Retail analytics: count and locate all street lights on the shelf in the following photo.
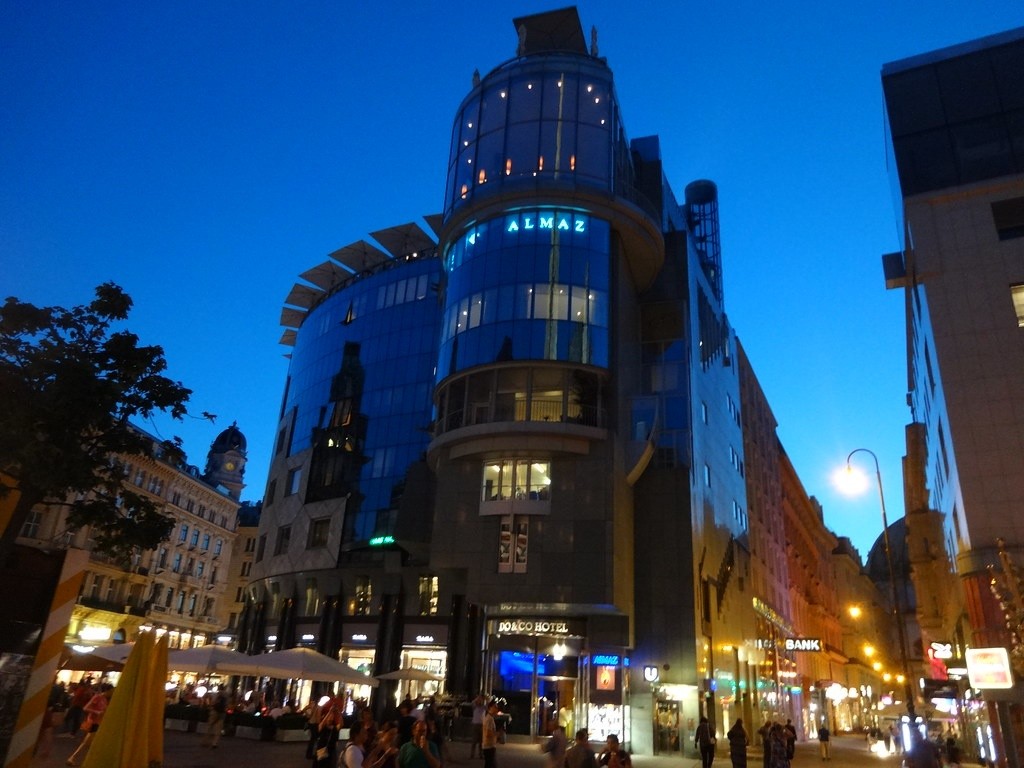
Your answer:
[838,448,922,751]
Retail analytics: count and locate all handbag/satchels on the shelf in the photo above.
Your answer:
[707,723,717,744]
[787,750,793,759]
[317,747,329,761]
[88,723,99,732]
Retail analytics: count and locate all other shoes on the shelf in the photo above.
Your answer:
[827,758,830,761]
[65,761,81,766]
[212,746,217,749]
[822,758,825,761]
[200,743,209,748]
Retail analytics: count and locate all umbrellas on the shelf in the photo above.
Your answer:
[59,642,443,687]
[81,628,165,768]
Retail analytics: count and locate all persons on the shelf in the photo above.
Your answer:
[863,723,944,754]
[202,684,499,768]
[51,679,115,768]
[694,716,797,768]
[540,704,632,768]
[818,724,832,761]
